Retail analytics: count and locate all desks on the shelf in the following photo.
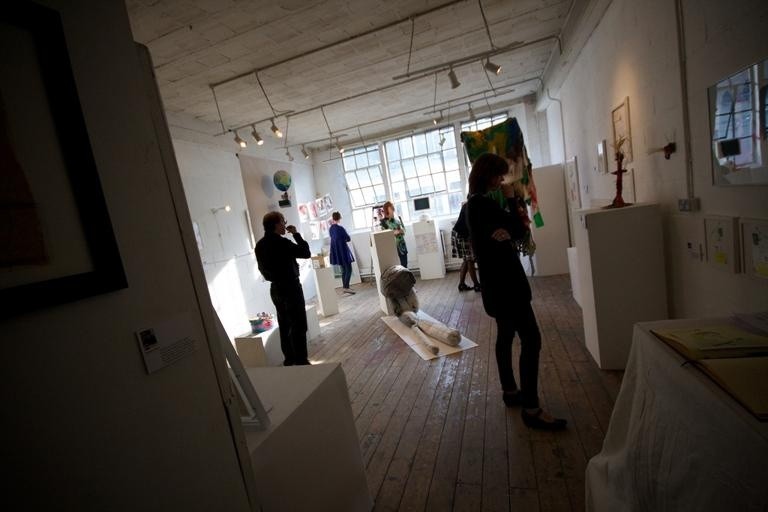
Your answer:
[627,315,766,510]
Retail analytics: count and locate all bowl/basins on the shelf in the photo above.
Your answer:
[249,316,273,335]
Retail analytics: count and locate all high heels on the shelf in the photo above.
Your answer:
[521,410,567,433]
[503,390,525,408]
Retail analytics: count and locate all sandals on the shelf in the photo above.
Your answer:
[474,284,481,292]
[458,283,474,292]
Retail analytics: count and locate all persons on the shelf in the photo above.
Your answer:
[450,203,481,292]
[254,211,311,366]
[329,212,356,294]
[380,202,408,268]
[464,154,567,431]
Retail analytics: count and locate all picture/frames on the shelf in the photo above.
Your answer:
[0,0,130,329]
[610,95,633,164]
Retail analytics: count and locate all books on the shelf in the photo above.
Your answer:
[649,326,768,423]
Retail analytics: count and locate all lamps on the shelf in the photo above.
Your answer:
[431,100,482,129]
[206,68,294,148]
[278,104,350,162]
[436,121,450,147]
[442,1,503,89]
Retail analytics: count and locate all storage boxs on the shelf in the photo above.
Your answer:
[312,255,328,268]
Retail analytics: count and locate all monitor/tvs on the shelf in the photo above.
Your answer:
[412,196,431,212]
